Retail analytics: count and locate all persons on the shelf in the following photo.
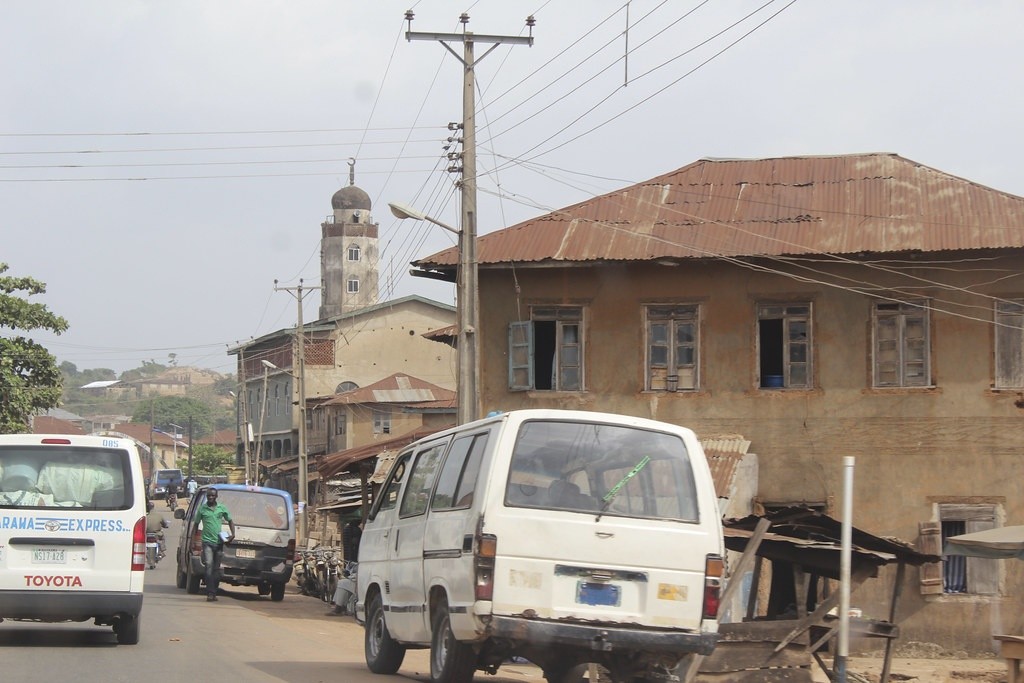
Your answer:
[189,487,235,602]
[164,477,179,507]
[145,502,169,558]
[325,530,362,617]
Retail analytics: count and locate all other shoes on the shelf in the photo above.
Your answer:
[324,605,342,616]
[213,593,219,601]
[207,593,214,602]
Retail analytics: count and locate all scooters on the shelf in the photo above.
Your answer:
[293,542,358,615]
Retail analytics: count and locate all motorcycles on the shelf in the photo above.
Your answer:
[165,488,178,511]
[145,520,172,570]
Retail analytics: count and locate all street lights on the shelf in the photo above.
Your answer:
[262,357,309,540]
[390,198,474,428]
[228,391,250,484]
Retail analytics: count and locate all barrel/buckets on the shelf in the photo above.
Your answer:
[764,375,784,387]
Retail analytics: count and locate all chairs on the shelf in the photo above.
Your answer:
[0,476,34,492]
[91,489,124,508]
[545,480,580,507]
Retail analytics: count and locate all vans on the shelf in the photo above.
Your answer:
[174,483,297,602]
[0,432,154,645]
[146,469,183,499]
[339,407,729,683]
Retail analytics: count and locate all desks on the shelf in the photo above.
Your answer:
[994,634,1024,683]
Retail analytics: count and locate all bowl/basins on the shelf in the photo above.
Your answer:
[850,608,862,617]
